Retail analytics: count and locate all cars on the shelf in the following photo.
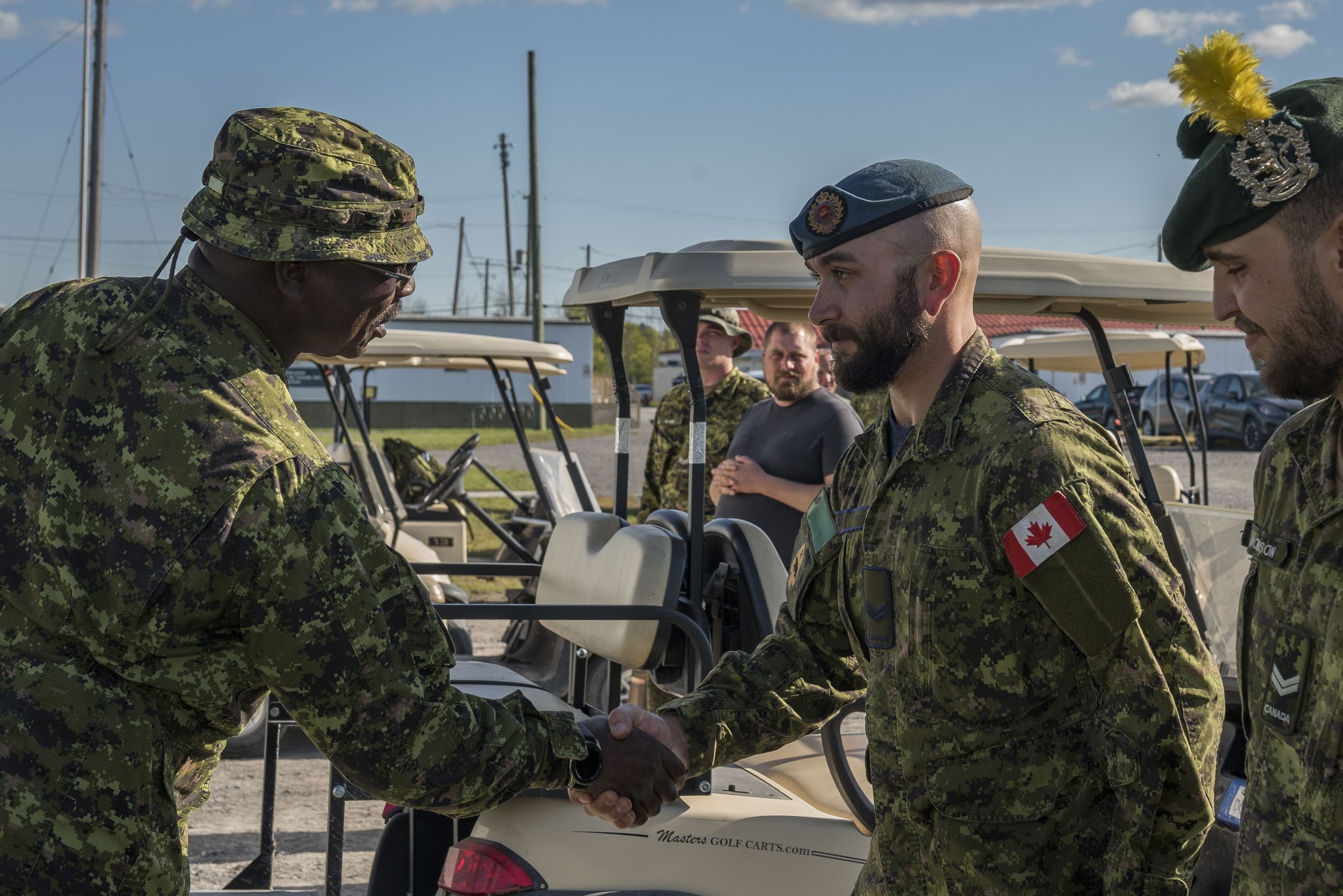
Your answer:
[1071,384,1148,430]
[1138,372,1217,436]
[1185,370,1305,451]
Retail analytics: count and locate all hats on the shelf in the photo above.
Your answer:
[181,107,434,265]
[697,305,754,359]
[1159,31,1343,273]
[790,159,975,259]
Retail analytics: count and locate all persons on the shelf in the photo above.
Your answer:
[1163,30,1343,896]
[635,291,889,570]
[568,160,1226,895]
[0,108,685,896]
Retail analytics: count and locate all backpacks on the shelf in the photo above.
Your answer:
[383,437,447,505]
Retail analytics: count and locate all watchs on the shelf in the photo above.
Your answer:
[570,721,605,793]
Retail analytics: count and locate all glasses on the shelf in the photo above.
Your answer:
[343,261,418,290]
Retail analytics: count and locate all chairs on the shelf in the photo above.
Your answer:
[445,505,790,724]
[734,699,882,840]
[330,439,469,565]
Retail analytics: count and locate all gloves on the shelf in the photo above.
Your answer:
[571,714,688,828]
